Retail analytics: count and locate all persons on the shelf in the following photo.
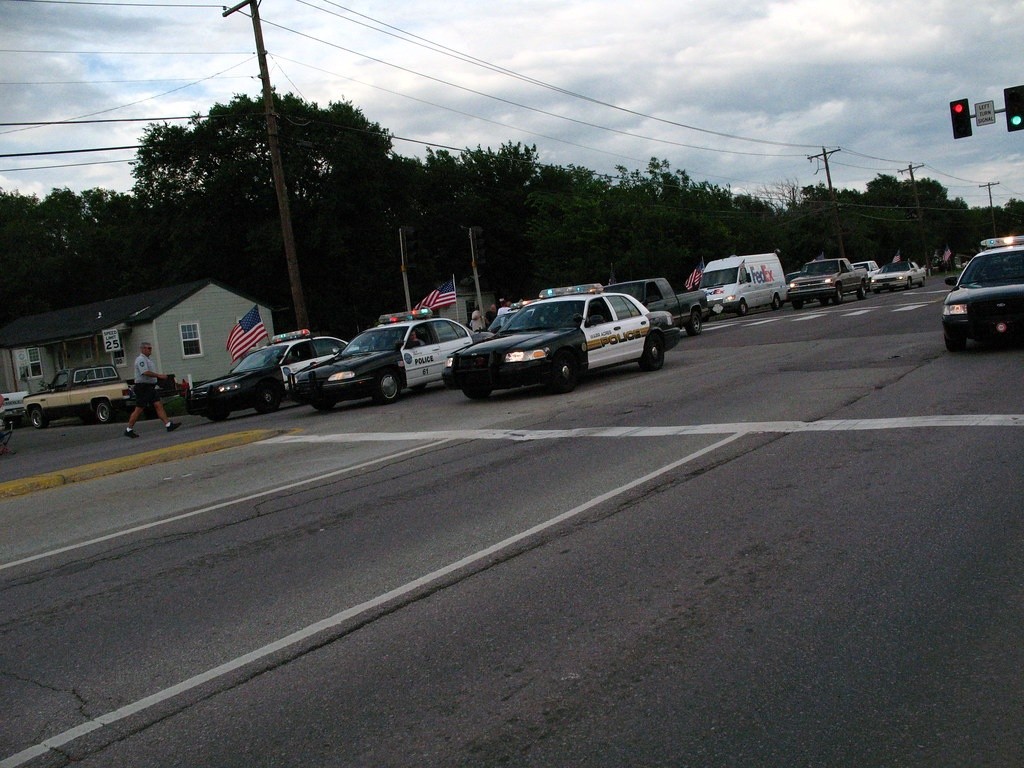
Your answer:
[123,339,182,438]
[469,300,512,331]
[408,329,425,346]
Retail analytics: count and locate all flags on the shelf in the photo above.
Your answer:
[684,260,705,291]
[413,279,456,311]
[942,244,951,263]
[891,250,900,263]
[226,304,268,365]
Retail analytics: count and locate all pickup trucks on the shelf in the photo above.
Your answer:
[0,390,29,427]
[23,364,178,429]
[603,277,708,336]
[787,258,868,309]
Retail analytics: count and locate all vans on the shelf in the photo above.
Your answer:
[700,252,788,318]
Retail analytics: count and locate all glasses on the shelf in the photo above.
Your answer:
[144,347,151,349]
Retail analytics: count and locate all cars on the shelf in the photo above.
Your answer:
[943,245,1024,351]
[851,261,881,291]
[188,336,371,422]
[296,318,495,410]
[442,293,681,399]
[784,271,801,302]
[869,261,926,293]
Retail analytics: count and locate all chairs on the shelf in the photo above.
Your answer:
[384,336,399,347]
[418,333,431,345]
[982,266,1004,282]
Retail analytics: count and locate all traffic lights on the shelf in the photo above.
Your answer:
[1004,85,1024,132]
[950,98,973,139]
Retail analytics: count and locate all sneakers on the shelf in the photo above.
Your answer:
[167,422,182,432]
[124,430,139,438]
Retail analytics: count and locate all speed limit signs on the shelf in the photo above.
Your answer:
[102,330,121,352]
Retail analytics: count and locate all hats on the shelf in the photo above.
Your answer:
[499,298,507,302]
